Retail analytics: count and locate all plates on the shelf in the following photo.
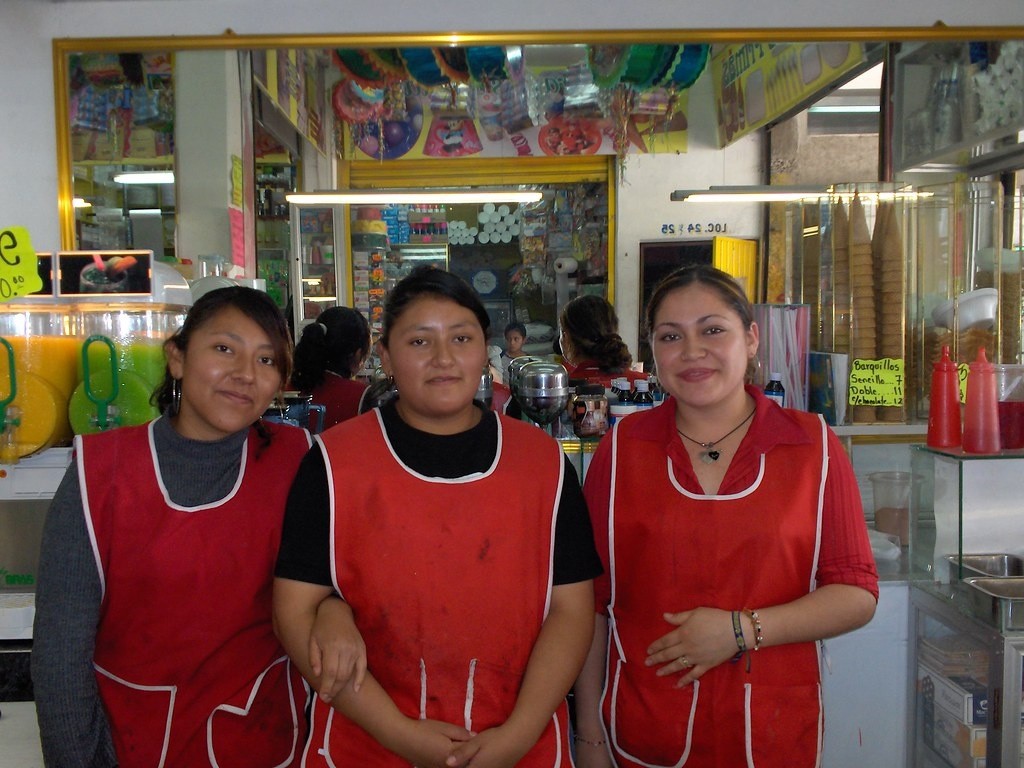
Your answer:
[973,247,1020,273]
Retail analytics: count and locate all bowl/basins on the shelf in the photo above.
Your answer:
[931,288,999,335]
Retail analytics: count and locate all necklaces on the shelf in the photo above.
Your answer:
[676,408,756,465]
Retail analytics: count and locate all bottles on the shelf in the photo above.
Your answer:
[962,346,1000,454]
[615,380,668,407]
[927,345,962,448]
[611,377,627,393]
[764,372,785,408]
[255,173,290,216]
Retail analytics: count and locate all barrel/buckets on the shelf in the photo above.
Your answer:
[320,245,333,264]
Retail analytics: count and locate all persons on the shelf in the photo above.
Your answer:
[580,264,879,768]
[269,263,605,768]
[30,286,368,768]
[280,294,653,442]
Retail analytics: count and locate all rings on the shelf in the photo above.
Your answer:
[682,655,691,669]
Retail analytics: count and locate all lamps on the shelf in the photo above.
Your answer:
[285,190,543,205]
[669,184,920,204]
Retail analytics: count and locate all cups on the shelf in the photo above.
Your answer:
[198,255,222,277]
[79,261,128,293]
[868,471,924,546]
[993,365,1024,449]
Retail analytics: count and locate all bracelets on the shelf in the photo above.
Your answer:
[732,609,762,672]
